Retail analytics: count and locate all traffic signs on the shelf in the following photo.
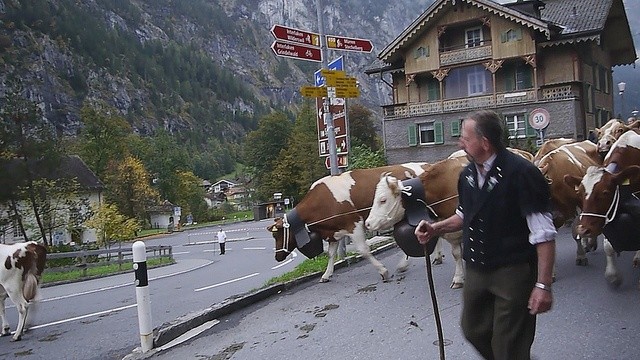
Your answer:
[529,108,551,130]
[313,55,350,169]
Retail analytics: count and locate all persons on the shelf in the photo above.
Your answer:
[619,119,625,124]
[415,109,559,360]
[214,228,227,255]
[628,117,635,125]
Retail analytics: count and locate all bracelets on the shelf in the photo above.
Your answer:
[535,282,552,291]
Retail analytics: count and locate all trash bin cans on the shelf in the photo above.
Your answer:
[254,204,267,221]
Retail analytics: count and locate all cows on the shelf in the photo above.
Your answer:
[533,118,640,290]
[364,144,536,290]
[0,239,46,340]
[266,160,445,284]
[446,148,534,162]
